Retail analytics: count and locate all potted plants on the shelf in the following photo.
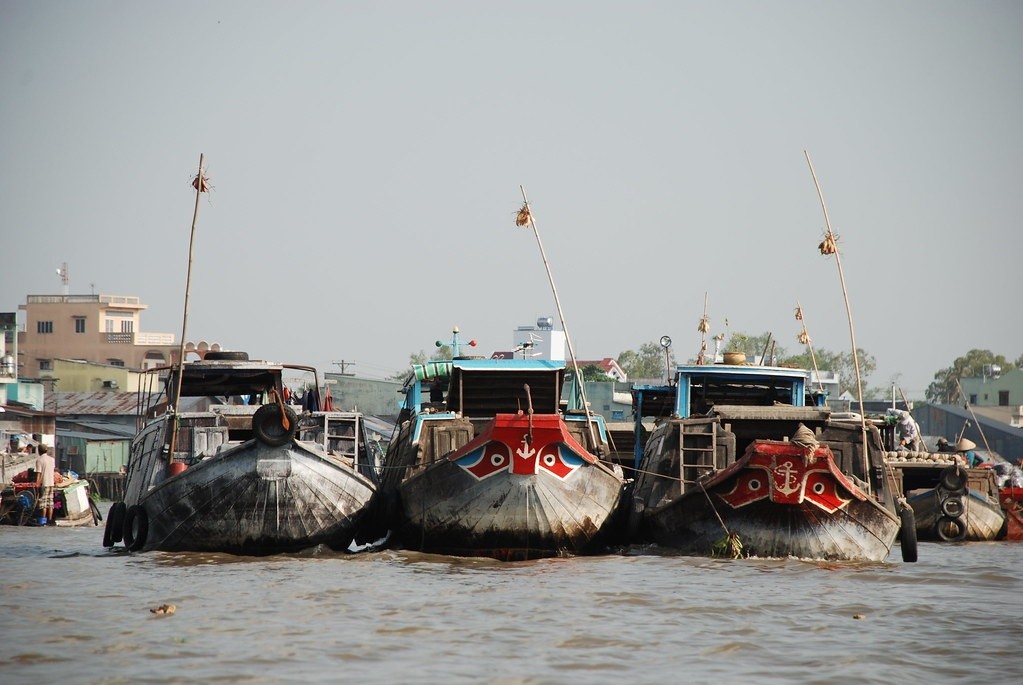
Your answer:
[723,318,748,365]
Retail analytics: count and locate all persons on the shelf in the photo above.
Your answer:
[36,444,55,525]
[935,438,984,470]
[886,408,920,451]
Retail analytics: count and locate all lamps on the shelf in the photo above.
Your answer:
[660,336,674,413]
[103,380,121,390]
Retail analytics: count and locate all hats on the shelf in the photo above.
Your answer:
[38,444,48,451]
[935,438,948,446]
[956,438,977,451]
[899,411,910,425]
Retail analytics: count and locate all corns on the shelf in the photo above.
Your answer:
[796,311,808,344]
[697,322,708,332]
[516,214,530,227]
[818,238,835,254]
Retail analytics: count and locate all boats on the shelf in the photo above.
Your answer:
[0,437,101,527]
[100,142,382,551]
[360,179,638,560]
[628,150,902,561]
[885,458,1005,544]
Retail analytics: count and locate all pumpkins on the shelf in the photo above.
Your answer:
[883,450,961,465]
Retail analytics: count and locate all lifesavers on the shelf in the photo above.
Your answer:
[898,509,918,563]
[934,465,970,541]
[250,402,300,447]
[102,501,150,550]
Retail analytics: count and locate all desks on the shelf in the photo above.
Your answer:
[874,420,896,452]
